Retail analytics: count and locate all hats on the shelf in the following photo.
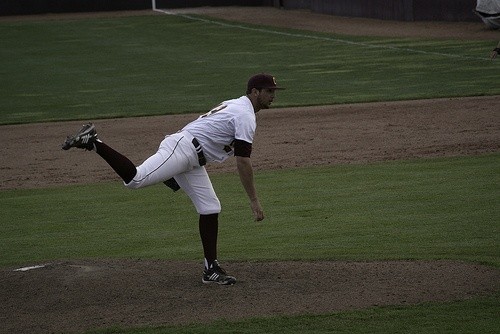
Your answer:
[248,72,286,89]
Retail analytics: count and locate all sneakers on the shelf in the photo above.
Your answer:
[201,257,237,286]
[62,121,98,151]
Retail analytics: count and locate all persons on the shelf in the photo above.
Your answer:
[62,73,286,285]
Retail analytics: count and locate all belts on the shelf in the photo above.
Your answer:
[192,138,206,166]
